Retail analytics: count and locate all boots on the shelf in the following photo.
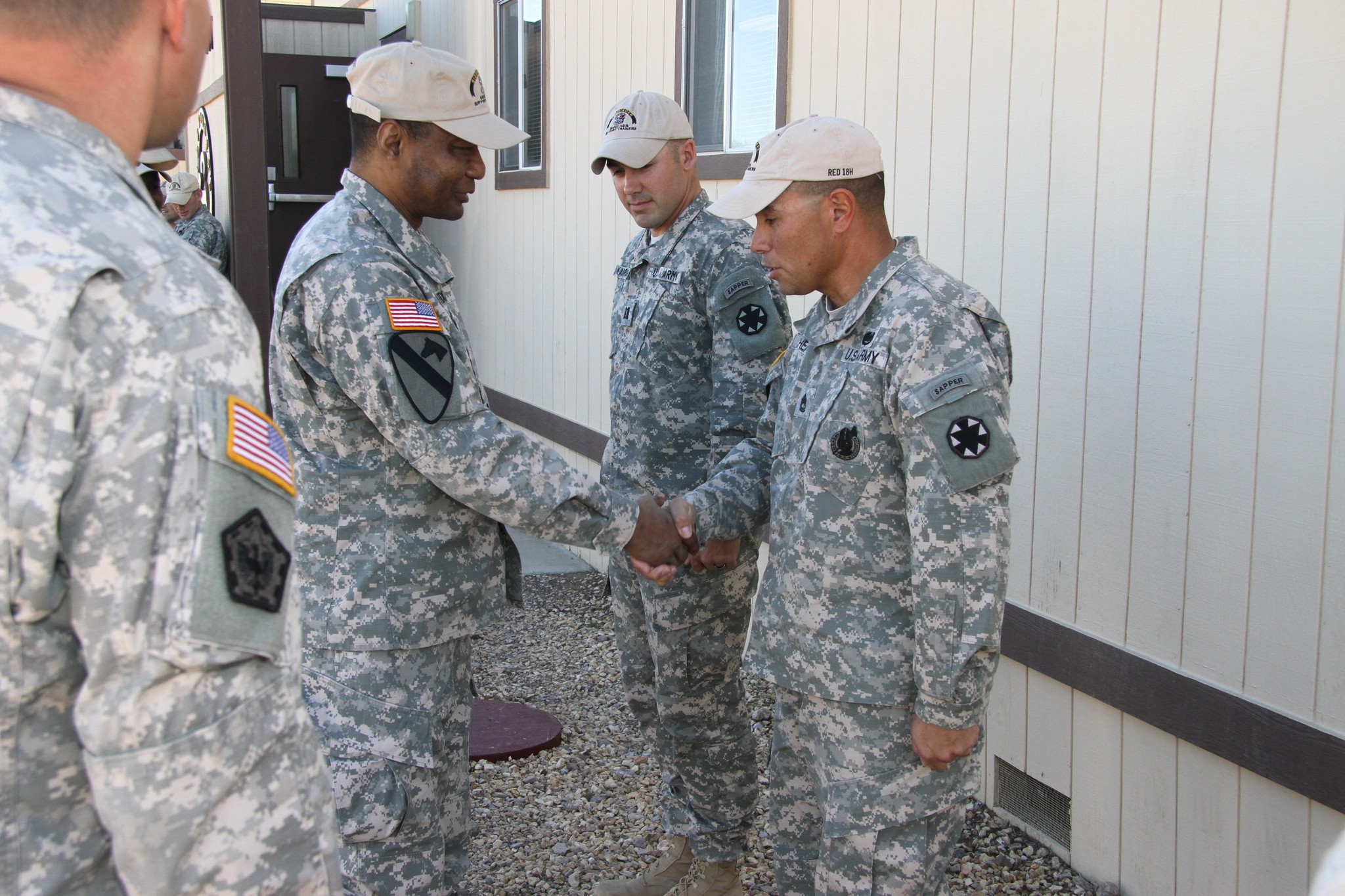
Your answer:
[593,828,693,896]
[661,859,743,896]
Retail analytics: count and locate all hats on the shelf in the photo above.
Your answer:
[344,40,536,148]
[708,116,884,220]
[586,89,693,175]
[164,173,198,206]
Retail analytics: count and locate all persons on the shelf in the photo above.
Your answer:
[627,113,1024,896]
[267,40,702,896]
[164,170,227,278]
[0,0,349,896]
[134,160,173,213]
[157,180,183,234]
[585,84,801,893]
